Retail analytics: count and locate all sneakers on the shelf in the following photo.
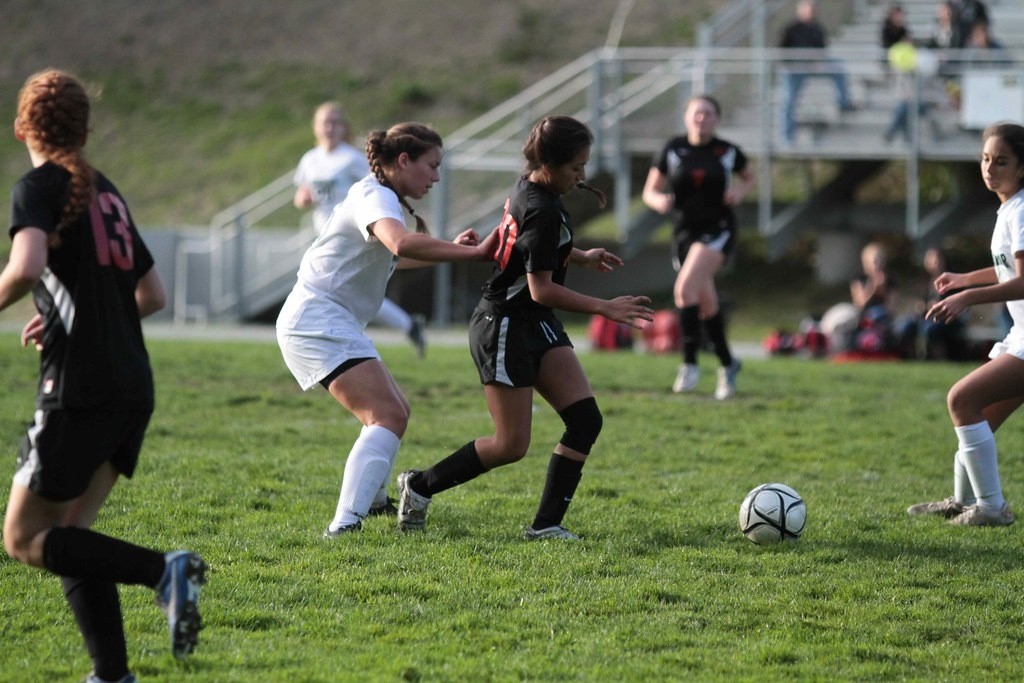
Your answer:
[396,468,433,532]
[714,358,740,400]
[673,362,700,392]
[949,504,1014,527]
[156,548,209,658]
[906,497,965,519]
[82,670,135,683]
[523,523,579,540]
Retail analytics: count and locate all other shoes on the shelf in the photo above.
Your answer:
[367,495,398,516]
[323,521,362,539]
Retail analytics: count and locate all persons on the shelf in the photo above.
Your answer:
[910,120,1022,527]
[404,115,656,546]
[0,67,211,683]
[276,122,499,542]
[297,0,1022,404]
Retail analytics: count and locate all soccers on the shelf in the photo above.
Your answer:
[738,482,807,547]
